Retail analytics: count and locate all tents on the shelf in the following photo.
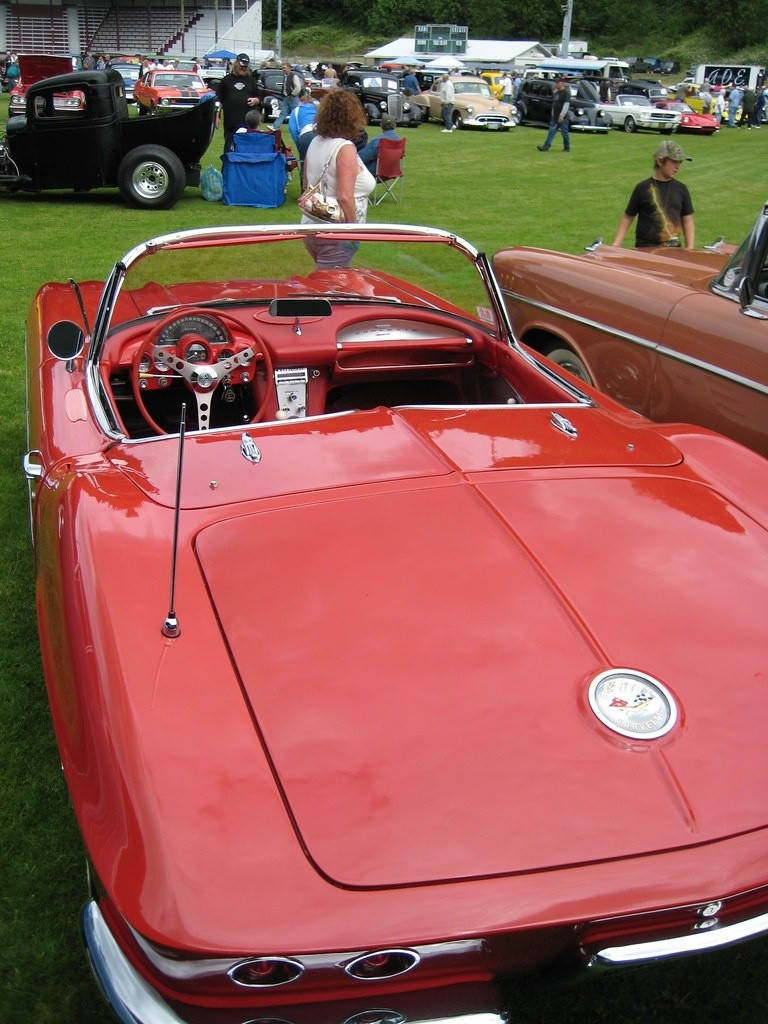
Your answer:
[203,50,239,70]
[383,54,426,66]
[425,56,464,68]
[229,49,282,72]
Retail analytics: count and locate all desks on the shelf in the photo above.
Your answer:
[293,160,304,178]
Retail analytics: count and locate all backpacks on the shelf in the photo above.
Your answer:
[290,72,306,94]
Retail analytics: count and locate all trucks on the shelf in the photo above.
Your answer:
[682,64,766,94]
[537,58,631,82]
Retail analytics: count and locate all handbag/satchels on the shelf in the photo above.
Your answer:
[281,140,298,171]
[200,165,223,202]
[297,184,350,225]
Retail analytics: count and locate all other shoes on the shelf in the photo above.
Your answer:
[537,146,549,152]
[451,125,457,129]
[722,123,763,131]
[560,149,570,153]
[441,129,452,133]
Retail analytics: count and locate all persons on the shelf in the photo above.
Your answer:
[300,92,376,269]
[4,51,19,93]
[82,51,112,71]
[441,74,457,133]
[214,53,264,173]
[447,67,482,77]
[387,65,421,96]
[305,64,311,72]
[597,79,611,104]
[142,57,233,76]
[324,63,349,78]
[496,71,568,105]
[537,78,570,152]
[288,95,402,194]
[231,110,293,194]
[266,63,301,131]
[612,140,695,249]
[675,78,768,130]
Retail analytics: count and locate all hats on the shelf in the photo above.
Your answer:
[410,69,415,74]
[653,141,695,162]
[175,58,181,62]
[553,76,562,80]
[236,53,250,67]
[381,115,396,129]
[694,78,767,93]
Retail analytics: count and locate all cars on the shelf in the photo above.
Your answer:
[57,53,227,91]
[253,69,321,123]
[338,70,423,128]
[133,70,221,117]
[411,76,520,132]
[604,56,681,75]
[9,53,86,118]
[595,94,682,135]
[109,64,142,100]
[523,69,624,100]
[291,61,514,100]
[618,79,768,124]
[491,201,767,461]
[1,69,218,210]
[29,221,767,1023]
[514,78,613,134]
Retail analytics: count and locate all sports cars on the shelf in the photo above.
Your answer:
[651,101,722,135]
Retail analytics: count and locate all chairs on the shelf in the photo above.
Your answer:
[220,132,295,208]
[624,101,633,106]
[368,137,407,207]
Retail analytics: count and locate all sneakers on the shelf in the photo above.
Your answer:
[267,125,276,132]
